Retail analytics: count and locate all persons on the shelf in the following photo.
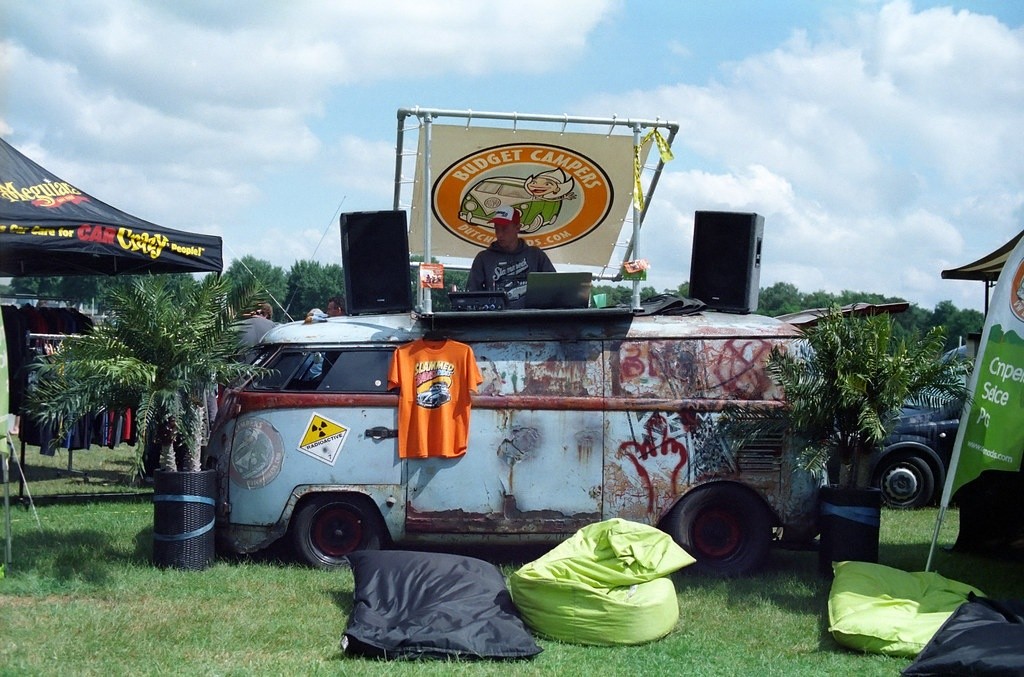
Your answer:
[465,206,557,309]
[326,297,345,317]
[237,302,277,348]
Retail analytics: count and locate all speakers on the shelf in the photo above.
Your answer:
[689,211,765,313]
[340,210,412,316]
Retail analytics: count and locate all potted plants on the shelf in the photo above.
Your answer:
[762,297,975,579]
[28,263,280,571]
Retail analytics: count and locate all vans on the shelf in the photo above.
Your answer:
[199,302,910,572]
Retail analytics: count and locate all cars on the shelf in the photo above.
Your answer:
[827,340,967,512]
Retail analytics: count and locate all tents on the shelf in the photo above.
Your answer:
[0,137,223,331]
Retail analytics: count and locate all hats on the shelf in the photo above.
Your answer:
[487,205,522,227]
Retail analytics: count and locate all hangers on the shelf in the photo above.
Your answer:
[422,314,447,342]
[34,333,66,353]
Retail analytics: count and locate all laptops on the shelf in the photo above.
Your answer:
[524,272,592,311]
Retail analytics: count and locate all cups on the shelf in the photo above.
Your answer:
[593,294,606,308]
